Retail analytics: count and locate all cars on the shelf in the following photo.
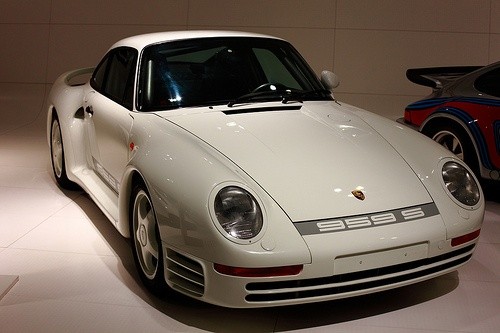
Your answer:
[397,59,499,182]
[45,29,486,309]
